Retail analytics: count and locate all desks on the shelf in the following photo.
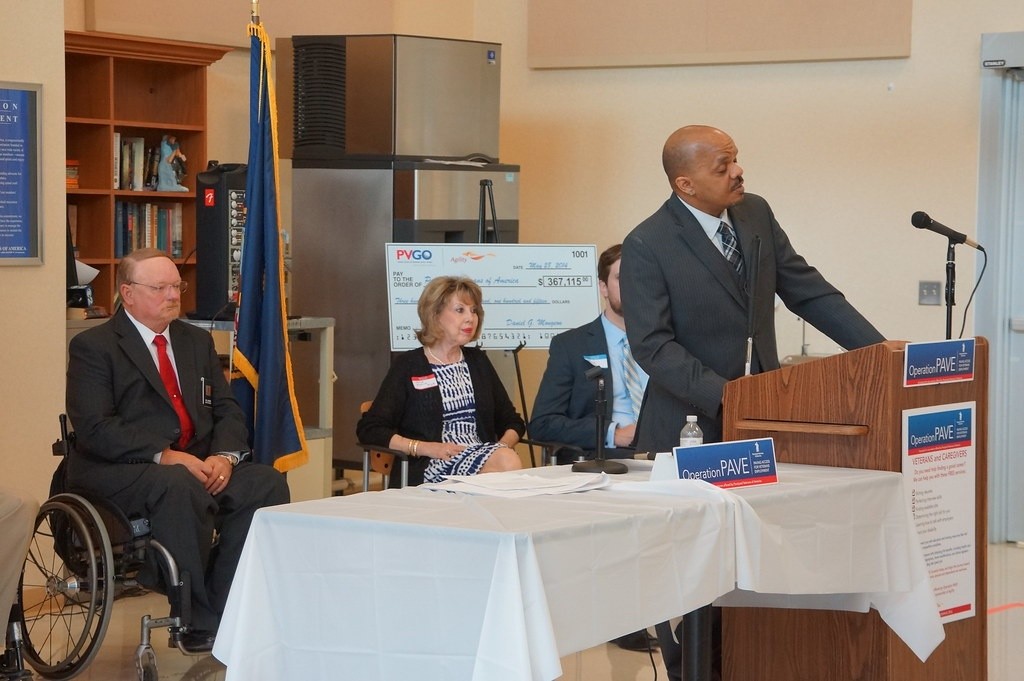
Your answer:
[257,458,903,681]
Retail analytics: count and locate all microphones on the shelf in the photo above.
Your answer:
[911,211,985,252]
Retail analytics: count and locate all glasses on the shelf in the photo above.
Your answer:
[128,281,188,294]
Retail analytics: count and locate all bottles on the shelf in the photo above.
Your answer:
[680,415,704,449]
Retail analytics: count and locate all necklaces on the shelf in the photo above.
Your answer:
[428,346,462,364]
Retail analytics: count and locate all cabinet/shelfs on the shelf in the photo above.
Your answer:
[66,317,336,582]
[64,30,234,319]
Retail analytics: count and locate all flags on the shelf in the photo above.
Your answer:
[233,21,309,474]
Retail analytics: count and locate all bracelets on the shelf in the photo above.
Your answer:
[408,440,420,456]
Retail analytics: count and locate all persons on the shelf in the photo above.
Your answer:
[620,125,912,681]
[527,244,659,653]
[65,247,287,655]
[158,135,186,188]
[356,276,524,490]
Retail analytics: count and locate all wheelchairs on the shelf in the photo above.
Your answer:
[14,412,222,681]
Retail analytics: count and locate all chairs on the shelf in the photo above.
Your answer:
[361,400,408,492]
[542,444,552,466]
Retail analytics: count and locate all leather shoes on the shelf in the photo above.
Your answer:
[167,629,217,651]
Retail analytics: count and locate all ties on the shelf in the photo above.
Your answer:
[152,335,196,453]
[622,336,645,424]
[717,220,744,275]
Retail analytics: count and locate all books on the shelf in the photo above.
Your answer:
[68,205,77,248]
[115,132,159,191]
[66,160,80,188]
[115,201,182,258]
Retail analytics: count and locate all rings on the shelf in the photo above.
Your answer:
[219,475,224,480]
[446,449,449,454]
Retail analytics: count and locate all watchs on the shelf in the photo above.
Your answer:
[221,454,237,466]
